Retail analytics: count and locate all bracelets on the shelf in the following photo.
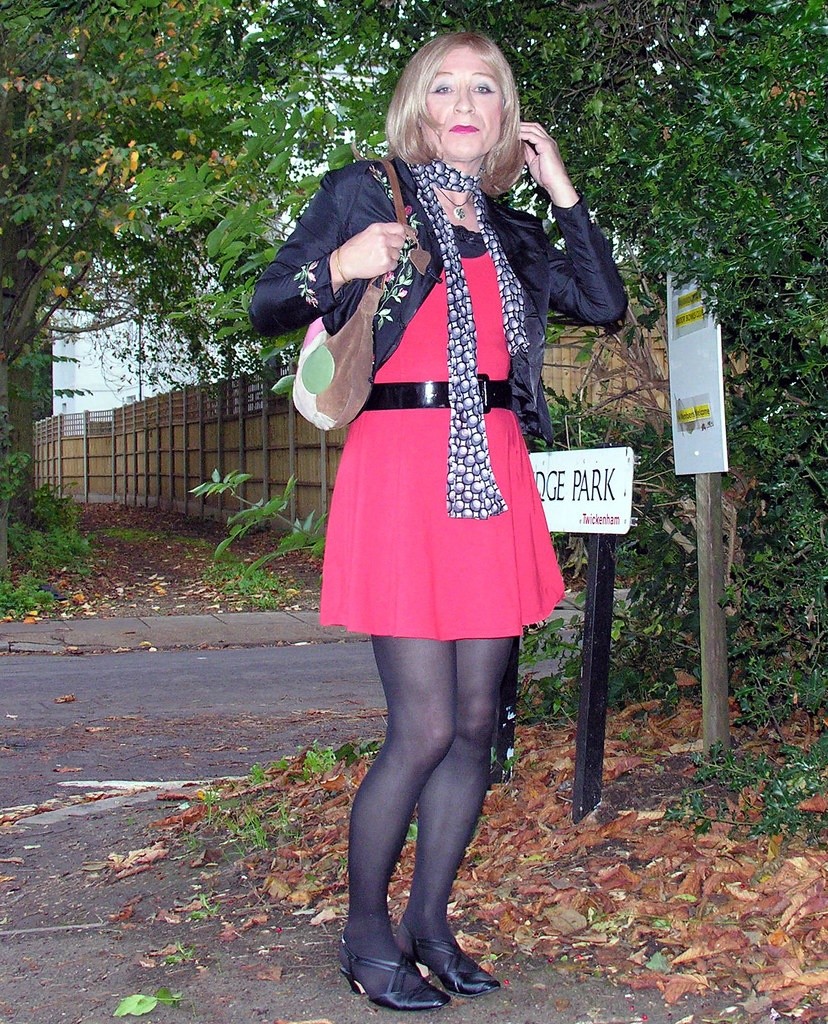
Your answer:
[335,246,354,285]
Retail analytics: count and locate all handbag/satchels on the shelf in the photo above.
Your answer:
[293,282,386,430]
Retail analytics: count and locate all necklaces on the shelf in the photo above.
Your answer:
[432,184,474,221]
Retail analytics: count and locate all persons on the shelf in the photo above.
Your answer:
[245,30,631,1013]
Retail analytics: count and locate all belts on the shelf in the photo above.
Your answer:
[364,374,513,413]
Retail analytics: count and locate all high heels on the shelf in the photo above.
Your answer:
[401,916,500,996]
[340,936,452,1010]
